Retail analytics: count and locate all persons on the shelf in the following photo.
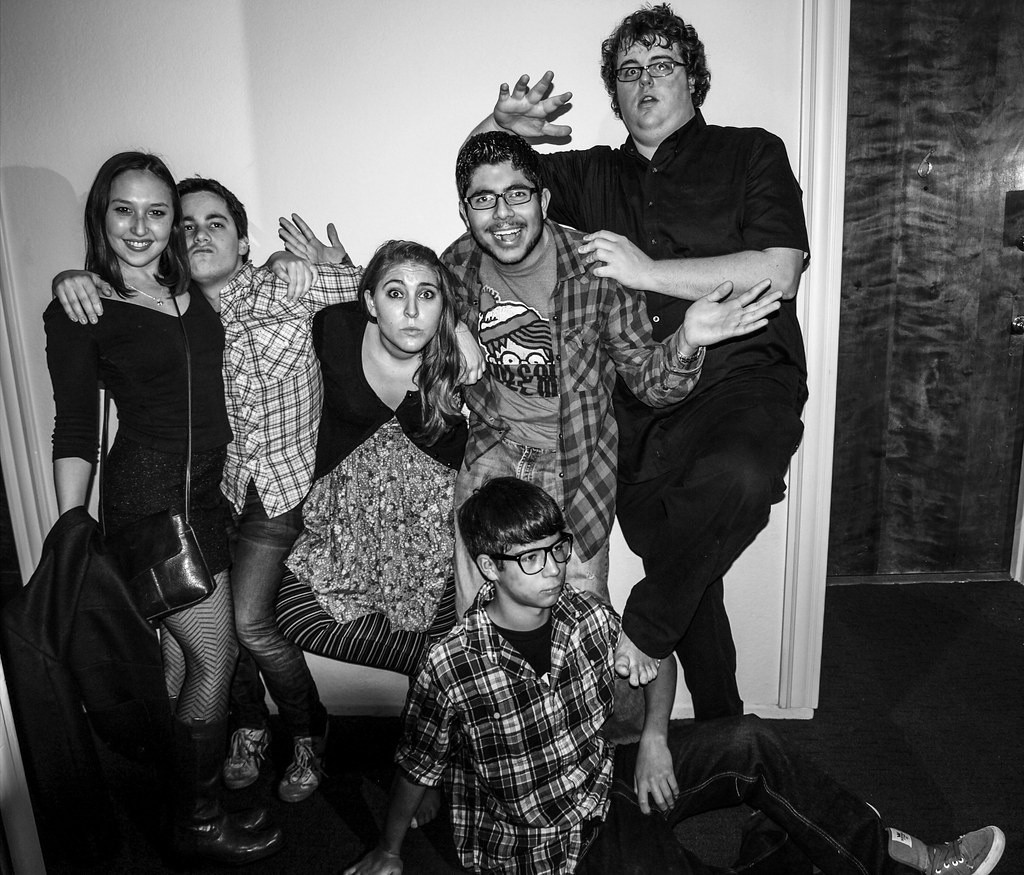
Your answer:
[43,4,1007,875]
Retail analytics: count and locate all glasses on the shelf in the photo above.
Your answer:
[490,532,574,576]
[615,61,687,82]
[463,188,538,210]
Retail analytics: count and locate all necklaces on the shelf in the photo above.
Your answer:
[120,278,165,307]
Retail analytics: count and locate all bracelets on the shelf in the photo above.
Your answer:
[676,345,704,365]
[376,844,400,859]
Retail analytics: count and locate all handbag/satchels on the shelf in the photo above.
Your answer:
[104,508,217,621]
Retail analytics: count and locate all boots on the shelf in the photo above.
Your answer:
[160,712,285,867]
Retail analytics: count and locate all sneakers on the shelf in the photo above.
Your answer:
[886,825,1006,875]
[278,717,330,803]
[750,798,882,821]
[222,727,271,790]
